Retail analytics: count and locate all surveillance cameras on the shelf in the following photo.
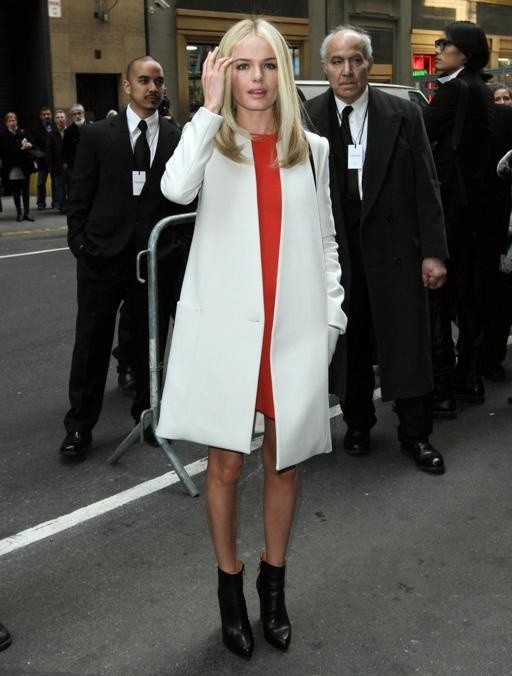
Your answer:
[154,0,170,9]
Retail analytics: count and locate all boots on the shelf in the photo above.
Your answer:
[217,562,254,660]
[257,552,292,653]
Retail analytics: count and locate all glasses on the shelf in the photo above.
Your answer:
[434,38,454,51]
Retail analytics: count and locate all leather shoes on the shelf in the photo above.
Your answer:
[59,429,94,457]
[117,364,141,391]
[399,439,445,474]
[343,425,373,455]
[434,390,457,420]
[460,370,486,403]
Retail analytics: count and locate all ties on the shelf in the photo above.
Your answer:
[133,123,151,170]
[339,107,362,230]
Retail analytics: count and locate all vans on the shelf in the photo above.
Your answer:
[293,77,433,112]
